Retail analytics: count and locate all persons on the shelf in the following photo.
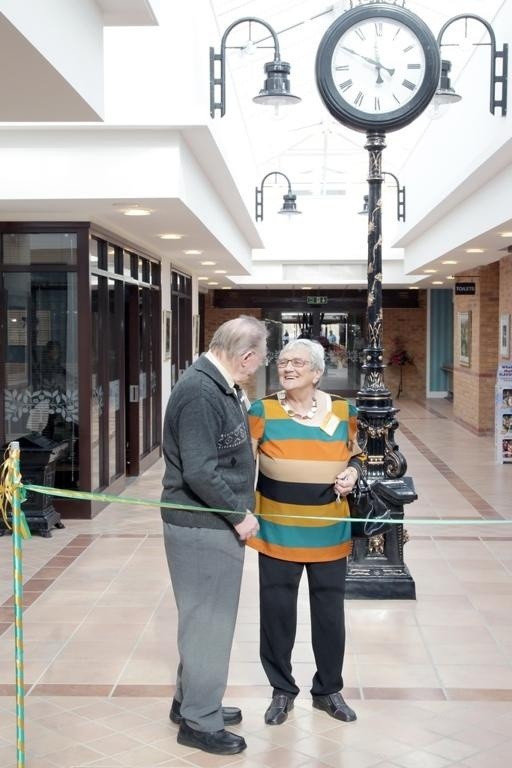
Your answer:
[328,329,336,342]
[28,339,79,446]
[157,313,269,756]
[248,335,364,725]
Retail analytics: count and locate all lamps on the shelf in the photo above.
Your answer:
[430,12,508,116]
[210,16,303,119]
[358,171,405,222]
[315,3,440,601]
[256,172,301,221]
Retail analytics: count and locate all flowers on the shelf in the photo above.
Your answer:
[387,336,416,366]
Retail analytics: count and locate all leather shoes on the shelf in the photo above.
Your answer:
[264,694,294,725]
[177,719,247,755]
[312,692,356,722]
[170,699,242,725]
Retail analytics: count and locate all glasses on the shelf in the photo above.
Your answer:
[277,358,309,368]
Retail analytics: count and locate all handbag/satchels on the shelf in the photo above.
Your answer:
[346,462,394,539]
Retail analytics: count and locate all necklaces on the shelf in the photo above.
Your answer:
[282,392,319,420]
